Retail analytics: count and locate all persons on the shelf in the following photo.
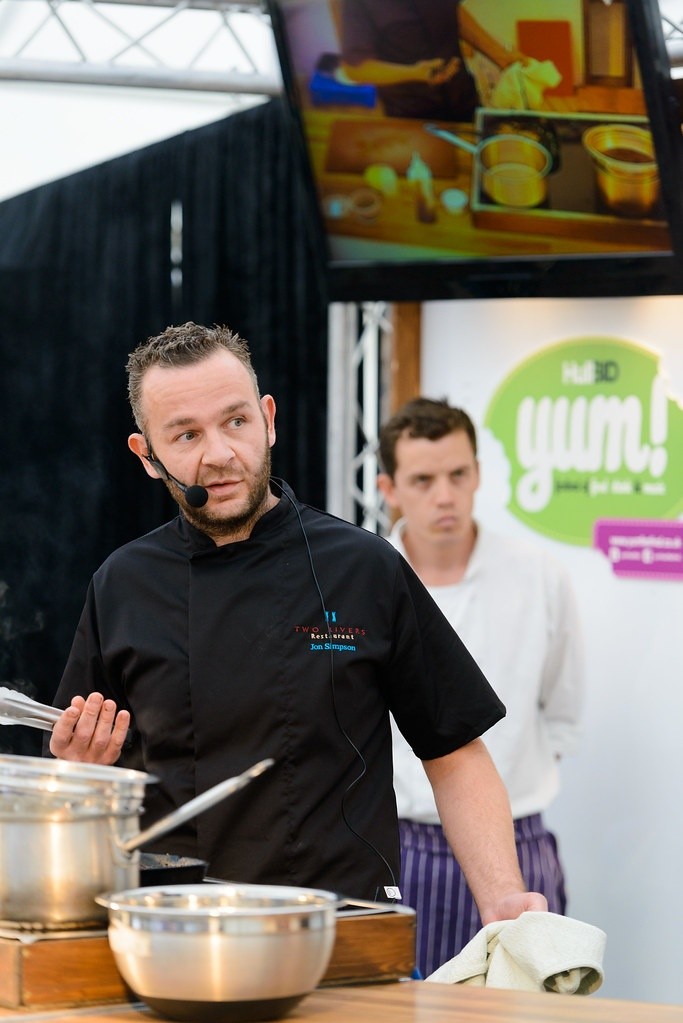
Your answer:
[378,398,589,991]
[328,0,529,123]
[48,321,549,934]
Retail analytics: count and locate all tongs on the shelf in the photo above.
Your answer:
[1,686,132,746]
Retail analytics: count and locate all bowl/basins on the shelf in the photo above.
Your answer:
[95,879,335,1022]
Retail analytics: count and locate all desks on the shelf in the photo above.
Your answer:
[0,980,683,1023]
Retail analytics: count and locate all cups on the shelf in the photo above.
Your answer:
[581,123,659,214]
[476,133,552,209]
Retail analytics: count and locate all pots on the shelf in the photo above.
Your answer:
[0,754,153,927]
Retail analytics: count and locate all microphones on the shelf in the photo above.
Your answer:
[142,443,209,507]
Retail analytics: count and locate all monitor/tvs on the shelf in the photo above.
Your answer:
[269,0,683,302]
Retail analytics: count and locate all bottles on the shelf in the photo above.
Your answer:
[406,150,438,224]
